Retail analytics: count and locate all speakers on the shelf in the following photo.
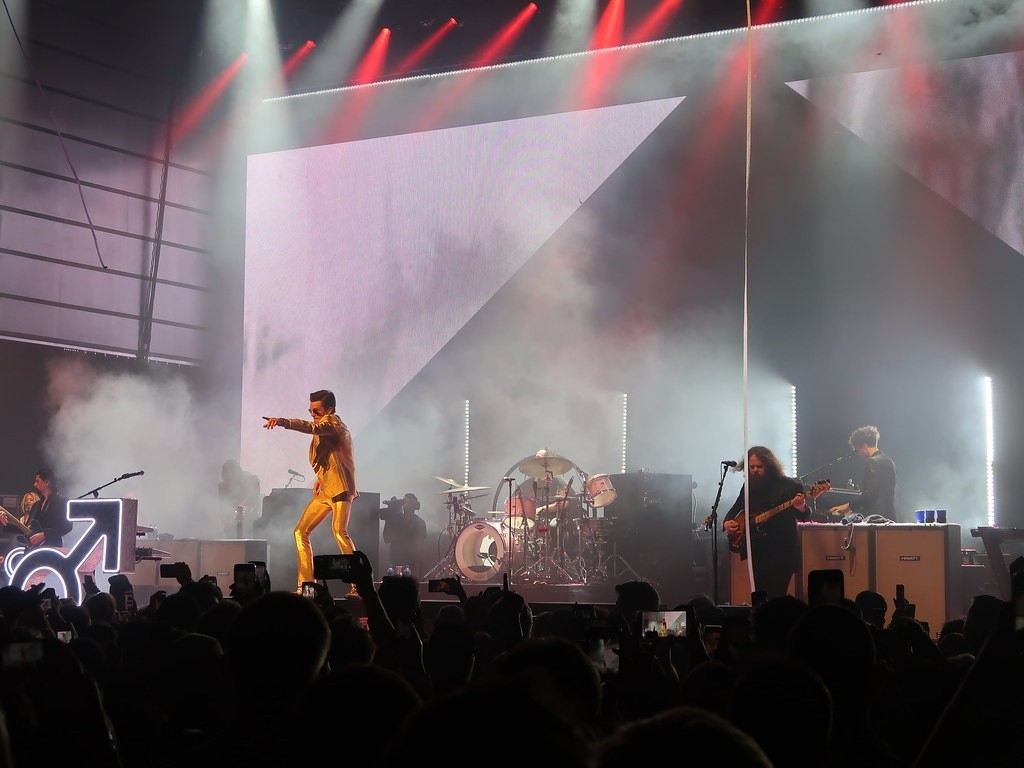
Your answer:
[125,537,267,595]
[730,523,961,641]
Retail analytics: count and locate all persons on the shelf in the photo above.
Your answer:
[216,459,261,540]
[660,617,666,636]
[0,492,40,565]
[0,551,1024,767]
[722,446,813,608]
[261,390,364,601]
[0,468,73,548]
[505,450,581,557]
[827,425,898,523]
[383,493,427,578]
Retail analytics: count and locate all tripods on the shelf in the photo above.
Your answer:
[512,451,641,584]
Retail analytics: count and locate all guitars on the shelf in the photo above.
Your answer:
[727,478,831,553]
[0,506,49,551]
[233,506,247,539]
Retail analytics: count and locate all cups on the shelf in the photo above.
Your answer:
[58,631,71,643]
[937,509,946,523]
[915,510,924,524]
[925,510,935,523]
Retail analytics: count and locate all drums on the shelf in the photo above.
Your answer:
[574,517,618,537]
[454,519,516,582]
[581,474,618,508]
[502,497,536,530]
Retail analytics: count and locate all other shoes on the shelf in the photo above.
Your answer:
[291,587,314,598]
[346,587,363,601]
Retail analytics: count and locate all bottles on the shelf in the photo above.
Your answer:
[148,523,158,540]
[387,564,411,577]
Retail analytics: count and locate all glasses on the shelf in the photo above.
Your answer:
[308,406,333,414]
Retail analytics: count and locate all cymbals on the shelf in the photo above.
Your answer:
[539,495,578,502]
[519,456,573,478]
[432,475,462,488]
[439,486,493,494]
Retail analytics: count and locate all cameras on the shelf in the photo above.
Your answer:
[56,630,73,643]
[41,598,52,611]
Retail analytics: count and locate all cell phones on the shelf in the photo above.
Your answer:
[313,554,352,579]
[234,564,256,595]
[428,580,450,592]
[248,560,265,589]
[587,626,620,676]
[160,564,176,578]
[638,609,693,640]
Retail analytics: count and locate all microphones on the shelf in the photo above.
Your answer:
[288,469,304,477]
[503,477,515,480]
[122,471,145,479]
[722,460,737,467]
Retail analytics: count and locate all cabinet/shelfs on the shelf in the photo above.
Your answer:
[124,541,159,587]
[874,528,961,639]
[801,529,872,602]
[731,551,799,605]
[198,538,267,598]
[156,539,201,586]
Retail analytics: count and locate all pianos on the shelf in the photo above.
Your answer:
[803,483,845,522]
[135,547,172,561]
